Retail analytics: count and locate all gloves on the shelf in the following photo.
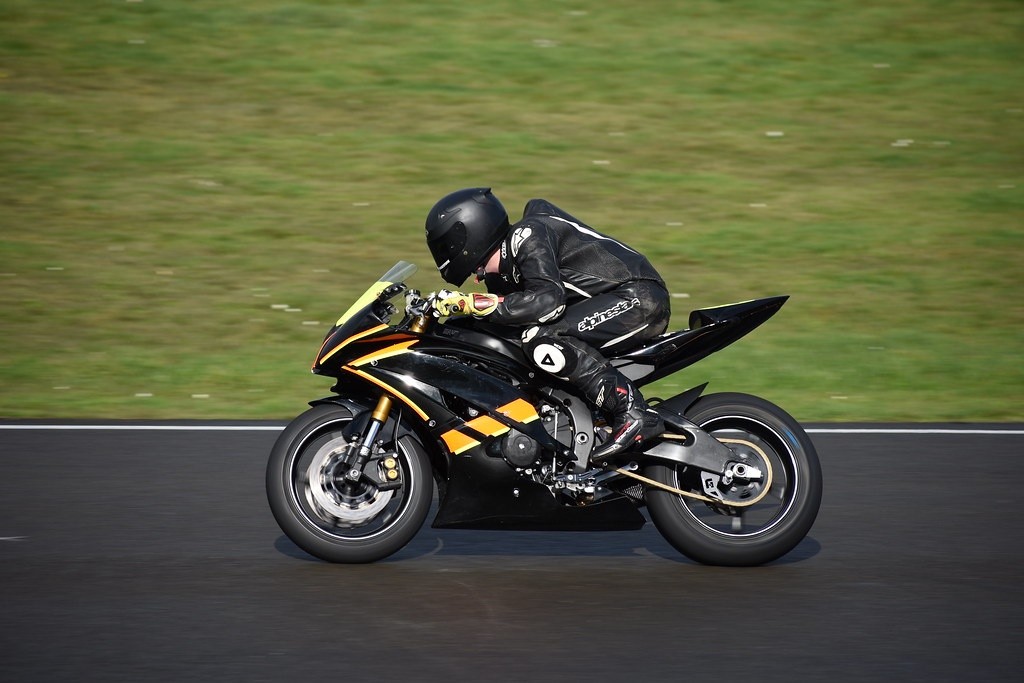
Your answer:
[431,288,498,324]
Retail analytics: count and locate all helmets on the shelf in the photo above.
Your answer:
[425,187,513,288]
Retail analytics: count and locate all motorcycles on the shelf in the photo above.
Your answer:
[264,261,825,566]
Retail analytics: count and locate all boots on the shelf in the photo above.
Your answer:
[580,363,665,463]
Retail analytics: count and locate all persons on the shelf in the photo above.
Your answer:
[420,187,672,462]
